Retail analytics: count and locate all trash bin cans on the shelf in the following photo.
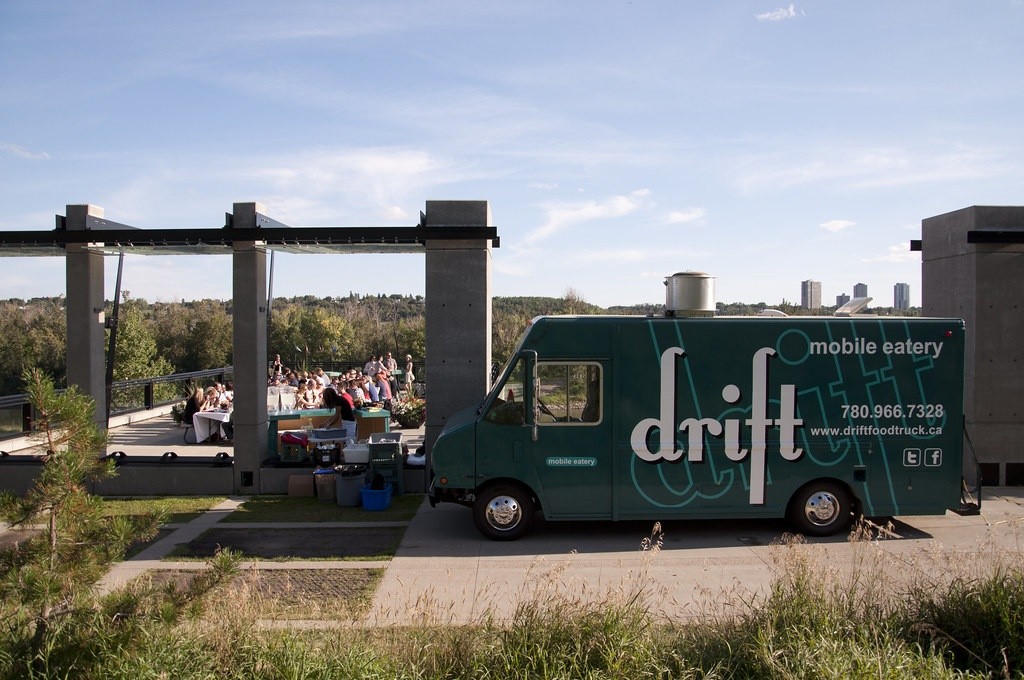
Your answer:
[334,465,368,506]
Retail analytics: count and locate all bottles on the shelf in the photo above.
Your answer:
[306,418,313,437]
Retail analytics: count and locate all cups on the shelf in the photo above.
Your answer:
[344,439,369,449]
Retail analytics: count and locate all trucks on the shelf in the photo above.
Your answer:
[429,309,982,538]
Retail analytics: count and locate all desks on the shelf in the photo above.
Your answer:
[270,407,392,439]
[267,385,298,411]
[192,409,232,444]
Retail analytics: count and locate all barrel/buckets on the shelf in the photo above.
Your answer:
[664,272,719,315]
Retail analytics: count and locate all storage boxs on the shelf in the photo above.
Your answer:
[279,428,404,509]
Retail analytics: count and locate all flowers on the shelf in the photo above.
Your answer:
[390,385,427,423]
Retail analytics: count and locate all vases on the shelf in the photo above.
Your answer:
[398,420,424,429]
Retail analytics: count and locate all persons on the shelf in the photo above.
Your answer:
[403,353,415,393]
[267,352,399,436]
[184,382,234,443]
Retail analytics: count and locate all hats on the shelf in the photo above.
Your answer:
[406,354,412,359]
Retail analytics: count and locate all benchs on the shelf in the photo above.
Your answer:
[94,451,235,495]
[0,450,50,495]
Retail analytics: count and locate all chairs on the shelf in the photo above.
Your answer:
[582,380,599,423]
